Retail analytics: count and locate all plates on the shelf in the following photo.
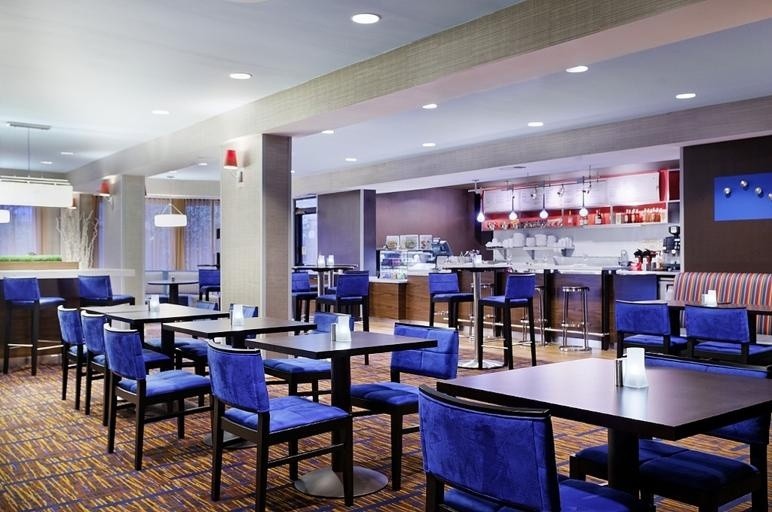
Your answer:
[511,230,574,249]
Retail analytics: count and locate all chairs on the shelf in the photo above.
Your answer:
[313,272,373,366]
[629,298,769,347]
[569,348,771,510]
[79,309,172,427]
[194,264,222,302]
[201,341,356,510]
[289,265,356,316]
[609,298,688,361]
[414,384,663,510]
[287,271,319,358]
[255,311,356,454]
[173,302,261,410]
[55,303,107,410]
[342,321,464,496]
[682,301,771,364]
[97,322,221,472]
[139,301,220,371]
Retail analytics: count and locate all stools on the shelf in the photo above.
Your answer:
[469,272,539,371]
[556,283,594,353]
[517,284,549,348]
[427,270,476,338]
[72,273,137,308]
[2,276,67,379]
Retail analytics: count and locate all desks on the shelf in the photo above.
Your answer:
[428,354,771,511]
[440,264,514,370]
[160,317,319,450]
[240,327,443,500]
[75,302,232,419]
[146,278,201,308]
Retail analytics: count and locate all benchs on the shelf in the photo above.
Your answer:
[670,271,771,347]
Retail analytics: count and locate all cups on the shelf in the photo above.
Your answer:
[623,347,648,390]
[474,255,483,263]
[667,285,675,304]
[335,314,353,342]
[150,295,161,312]
[232,304,245,325]
[706,289,718,307]
[316,254,335,268]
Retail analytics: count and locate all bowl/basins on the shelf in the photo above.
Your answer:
[485,238,512,249]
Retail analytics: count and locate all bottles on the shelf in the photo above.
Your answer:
[634,249,660,272]
[567,208,589,227]
[593,209,603,225]
[171,274,175,282]
[623,207,664,223]
[382,270,406,280]
[487,220,545,230]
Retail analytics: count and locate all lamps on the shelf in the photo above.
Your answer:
[96,179,114,207]
[505,190,519,220]
[576,182,589,218]
[0,121,74,208]
[224,149,244,183]
[536,187,552,221]
[154,200,188,228]
[474,190,488,223]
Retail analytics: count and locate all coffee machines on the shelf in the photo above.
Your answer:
[662,225,682,272]
